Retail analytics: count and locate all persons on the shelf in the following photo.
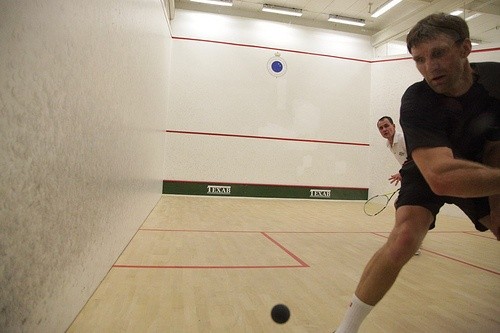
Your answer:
[377,116,422,255]
[334,13,500,333]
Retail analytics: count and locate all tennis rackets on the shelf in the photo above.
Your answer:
[362,186,402,218]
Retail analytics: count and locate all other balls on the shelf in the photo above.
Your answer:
[271,304,291,324]
